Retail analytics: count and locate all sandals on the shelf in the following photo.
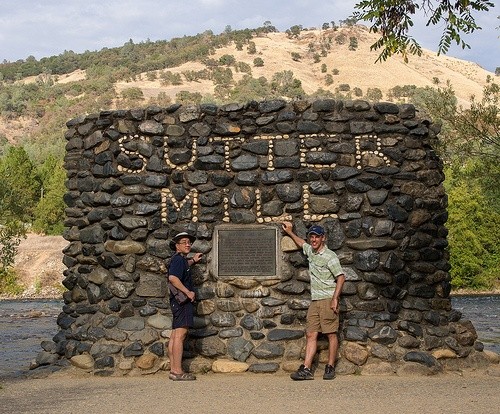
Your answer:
[173,372,196,381]
[169,372,175,379]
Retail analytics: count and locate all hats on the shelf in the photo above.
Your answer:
[170,231,196,251]
[308,224,324,236]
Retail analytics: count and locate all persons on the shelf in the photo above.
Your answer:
[282,221,346,380]
[167,232,203,381]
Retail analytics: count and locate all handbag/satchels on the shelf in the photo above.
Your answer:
[169,281,188,303]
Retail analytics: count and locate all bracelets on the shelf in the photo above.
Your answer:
[193,258,196,264]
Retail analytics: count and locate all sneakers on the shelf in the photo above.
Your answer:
[290,364,314,379]
[323,363,335,379]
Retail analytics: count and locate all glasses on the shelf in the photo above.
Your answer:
[176,240,193,245]
